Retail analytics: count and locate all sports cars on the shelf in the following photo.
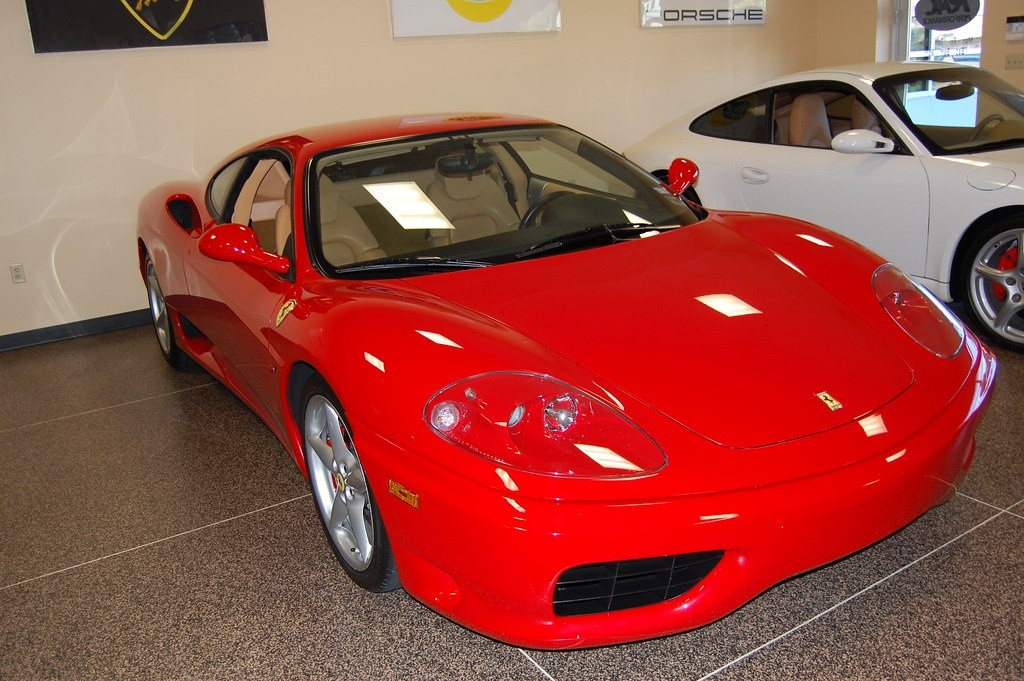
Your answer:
[135,112,1000,652]
[607,60,1024,358]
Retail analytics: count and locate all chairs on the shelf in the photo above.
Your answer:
[427,164,521,247]
[789,93,832,148]
[274,174,387,266]
[850,98,881,134]
